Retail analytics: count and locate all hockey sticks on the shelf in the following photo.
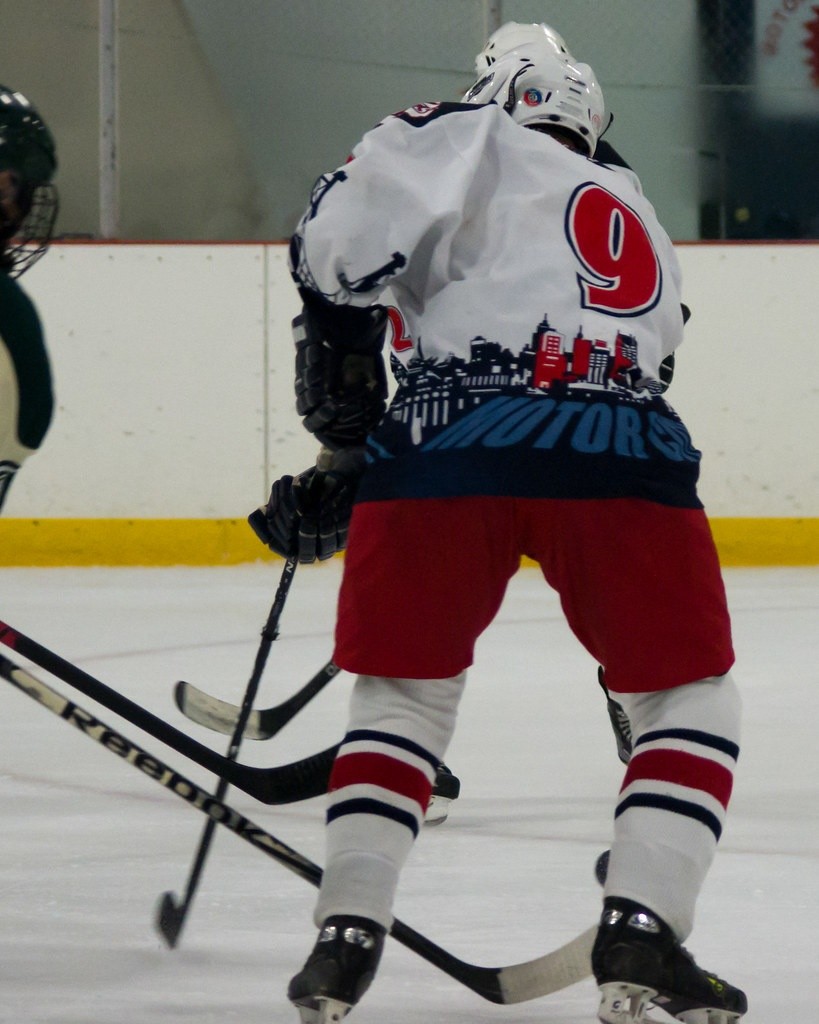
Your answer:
[0,656,601,1003]
[158,355,368,950]
[0,621,341,805]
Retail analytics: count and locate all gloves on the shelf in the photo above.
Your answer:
[290,318,388,448]
[248,466,351,561]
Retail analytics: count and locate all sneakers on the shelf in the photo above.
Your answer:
[592,899,749,1023]
[287,914,387,1022]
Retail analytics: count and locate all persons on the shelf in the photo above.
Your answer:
[0,88,58,534]
[244,19,754,1024]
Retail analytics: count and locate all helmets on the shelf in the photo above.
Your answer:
[463,20,607,157]
[0,85,62,287]
